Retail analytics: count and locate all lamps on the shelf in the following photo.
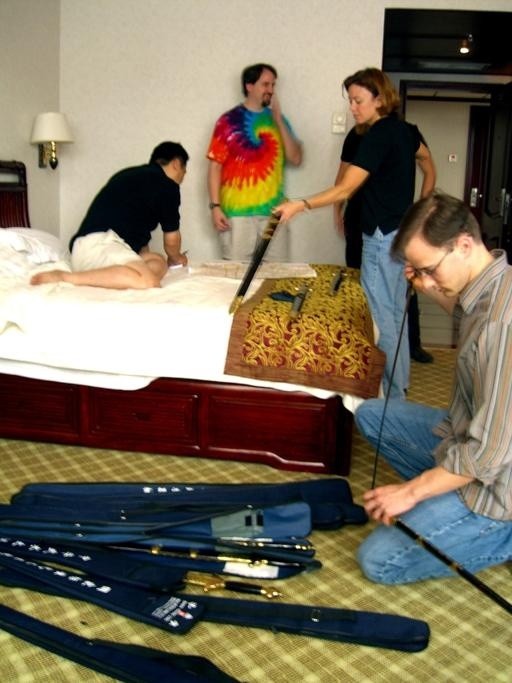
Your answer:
[32,112,73,168]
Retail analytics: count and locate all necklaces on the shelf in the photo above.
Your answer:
[29,141,189,289]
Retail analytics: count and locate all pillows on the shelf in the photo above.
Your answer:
[1,223,69,289]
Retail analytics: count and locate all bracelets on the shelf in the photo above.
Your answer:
[302,198,311,212]
[209,202,221,208]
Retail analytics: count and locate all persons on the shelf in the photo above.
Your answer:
[274,67,430,398]
[350,193,512,584]
[207,63,302,263]
[335,117,436,365]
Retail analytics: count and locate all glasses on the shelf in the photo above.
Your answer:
[413,251,450,276]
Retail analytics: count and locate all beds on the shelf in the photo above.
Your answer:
[0,159,387,478]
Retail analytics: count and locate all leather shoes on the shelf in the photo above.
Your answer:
[409,346,433,362]
